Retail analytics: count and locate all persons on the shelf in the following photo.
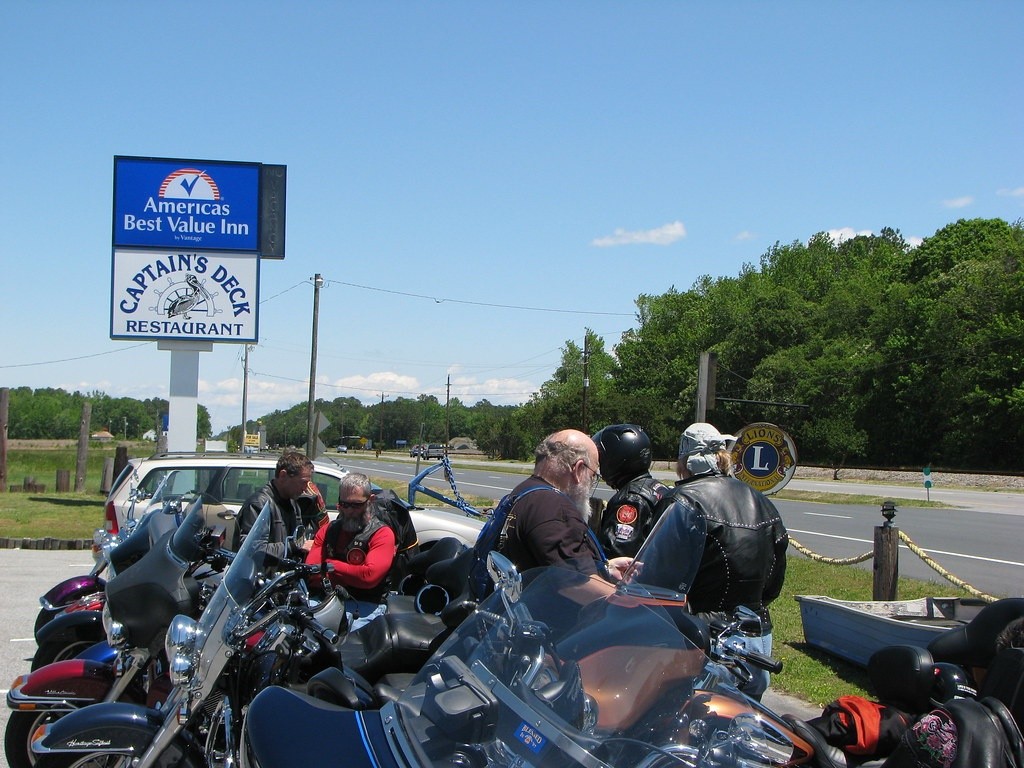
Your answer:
[806,599,1024,768]
[231,423,790,703]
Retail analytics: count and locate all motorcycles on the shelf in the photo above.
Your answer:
[2,500,1023,767]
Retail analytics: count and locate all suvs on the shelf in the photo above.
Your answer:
[90,451,487,564]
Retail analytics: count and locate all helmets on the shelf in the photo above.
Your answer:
[590,423,653,490]
[928,662,976,711]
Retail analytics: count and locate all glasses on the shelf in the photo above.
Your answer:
[575,460,602,483]
[338,496,369,509]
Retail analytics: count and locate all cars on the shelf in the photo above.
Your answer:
[410,445,426,458]
[422,443,445,460]
[337,445,347,454]
